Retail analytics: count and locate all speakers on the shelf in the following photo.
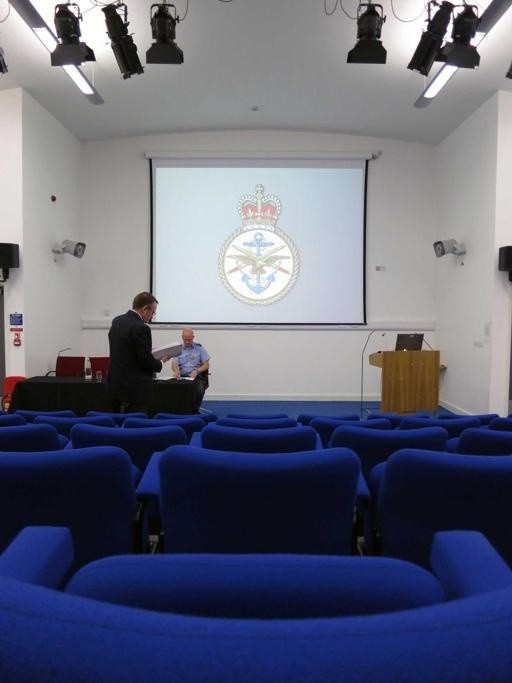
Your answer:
[0,243,19,269]
[499,246,512,271]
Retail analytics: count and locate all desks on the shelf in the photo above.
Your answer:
[7,376,202,414]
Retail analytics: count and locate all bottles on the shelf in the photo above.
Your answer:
[85,358,92,380]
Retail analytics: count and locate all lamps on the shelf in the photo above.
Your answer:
[345,0,512,108]
[0,0,185,106]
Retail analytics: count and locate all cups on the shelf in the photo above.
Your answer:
[96,370,103,382]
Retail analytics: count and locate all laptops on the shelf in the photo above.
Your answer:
[395,334,424,350]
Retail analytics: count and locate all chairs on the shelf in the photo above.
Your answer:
[43,355,86,378]
[202,361,210,391]
[87,356,110,379]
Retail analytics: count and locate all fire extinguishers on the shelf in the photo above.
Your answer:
[2,391,12,412]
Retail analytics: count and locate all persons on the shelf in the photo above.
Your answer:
[170,328,210,413]
[107,292,168,411]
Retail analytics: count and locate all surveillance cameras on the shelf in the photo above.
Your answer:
[53,239,87,259]
[433,239,465,258]
